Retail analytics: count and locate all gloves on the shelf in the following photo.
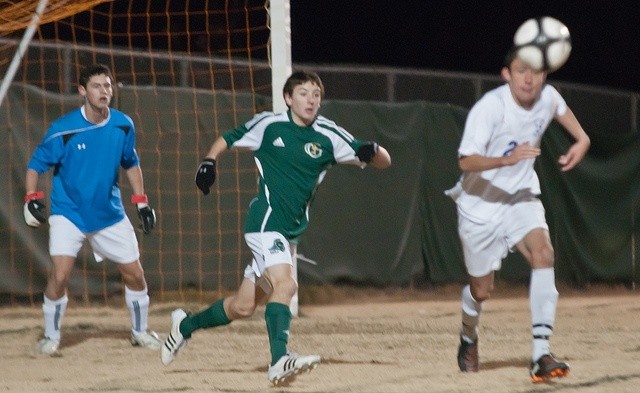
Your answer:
[355,141,377,170]
[23,198,46,228]
[136,202,157,233]
[194,158,217,194]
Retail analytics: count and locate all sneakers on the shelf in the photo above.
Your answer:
[128,327,163,350]
[38,336,60,354]
[268,351,322,386]
[160,307,189,366]
[458,328,479,371]
[529,354,568,382]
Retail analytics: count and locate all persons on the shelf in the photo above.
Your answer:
[445,49,592,385]
[92,31,116,40]
[160,71,392,387]
[23,64,163,353]
